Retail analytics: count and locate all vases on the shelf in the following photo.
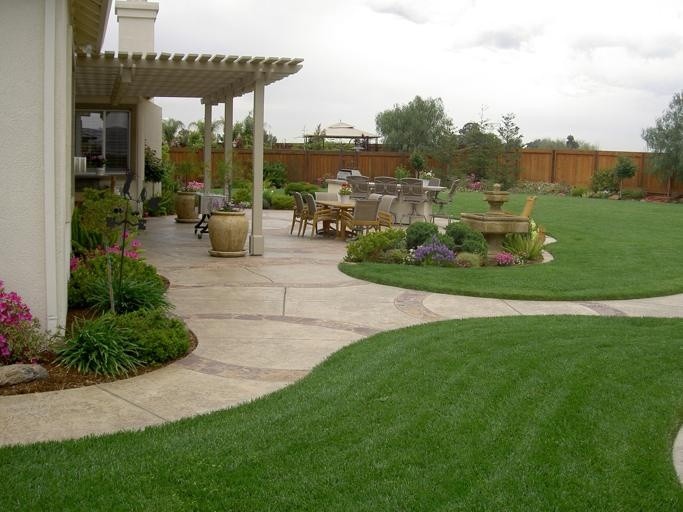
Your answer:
[173,191,201,223]
[206,211,249,257]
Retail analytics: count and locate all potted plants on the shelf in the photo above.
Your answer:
[94,158,112,176]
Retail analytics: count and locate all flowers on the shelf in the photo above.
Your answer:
[180,178,204,193]
[211,196,246,212]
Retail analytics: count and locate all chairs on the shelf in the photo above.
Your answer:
[289,175,461,242]
